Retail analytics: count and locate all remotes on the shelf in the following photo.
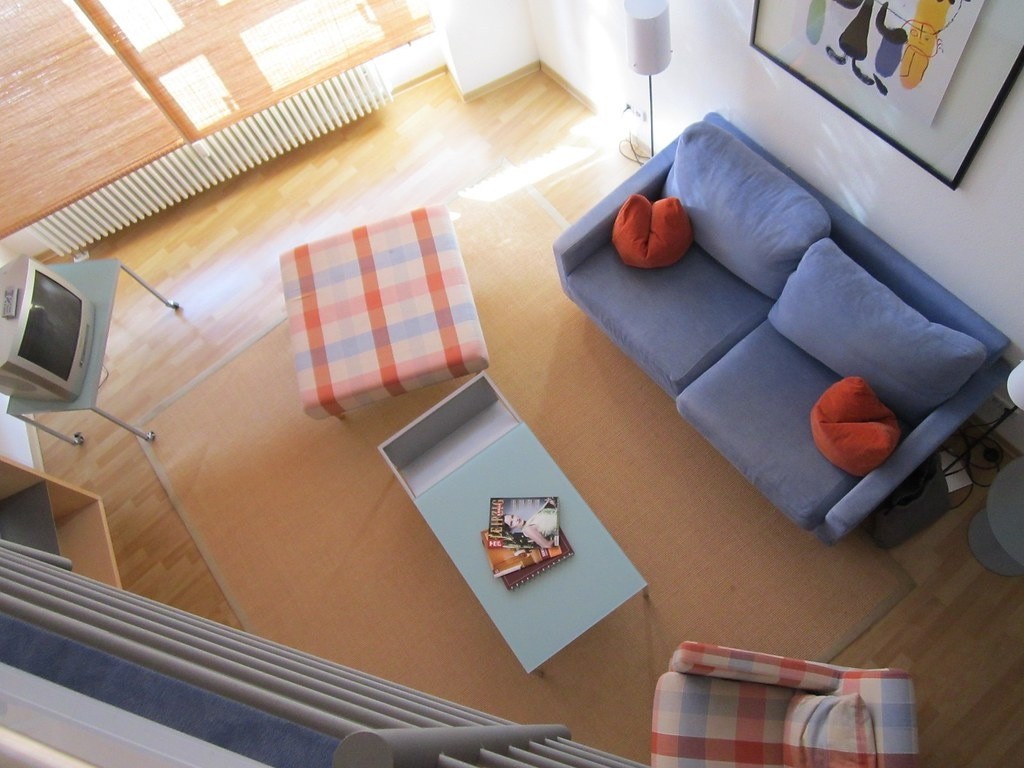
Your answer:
[2,288,17,318]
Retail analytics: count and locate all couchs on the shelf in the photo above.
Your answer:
[550,111,1013,546]
[650,641,920,768]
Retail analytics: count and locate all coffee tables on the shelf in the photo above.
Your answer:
[377,371,648,674]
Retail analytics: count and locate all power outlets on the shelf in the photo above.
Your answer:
[624,103,645,121]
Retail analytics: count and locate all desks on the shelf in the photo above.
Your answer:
[6,257,179,445]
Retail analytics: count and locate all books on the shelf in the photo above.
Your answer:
[480,497,575,592]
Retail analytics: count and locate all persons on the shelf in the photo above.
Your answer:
[498,498,558,548]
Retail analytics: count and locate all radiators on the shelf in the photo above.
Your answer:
[24,61,395,259]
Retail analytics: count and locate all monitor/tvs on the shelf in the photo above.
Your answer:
[0,255,95,403]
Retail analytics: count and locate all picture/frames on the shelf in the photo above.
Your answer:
[749,0,1024,192]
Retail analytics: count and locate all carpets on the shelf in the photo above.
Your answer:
[132,184,920,767]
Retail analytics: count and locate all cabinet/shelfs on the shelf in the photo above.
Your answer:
[0,455,123,591]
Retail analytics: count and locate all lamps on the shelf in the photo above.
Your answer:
[942,359,1024,476]
[624,0,673,157]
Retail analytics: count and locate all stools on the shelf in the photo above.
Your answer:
[280,203,489,419]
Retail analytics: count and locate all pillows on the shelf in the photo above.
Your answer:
[612,194,694,268]
[811,376,901,475]
[661,120,832,299]
[768,238,989,424]
